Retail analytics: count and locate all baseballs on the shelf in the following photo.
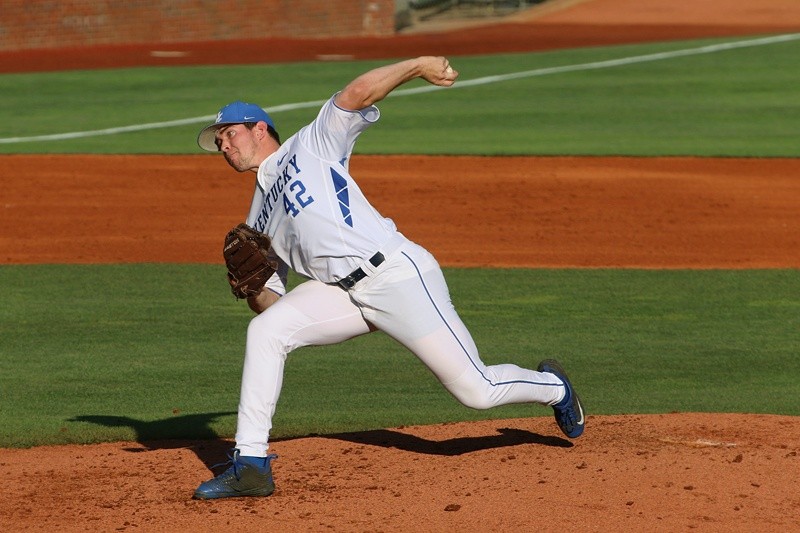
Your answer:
[446,65,453,74]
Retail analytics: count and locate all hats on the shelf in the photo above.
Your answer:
[197,101,275,152]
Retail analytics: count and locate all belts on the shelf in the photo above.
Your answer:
[337,251,385,290]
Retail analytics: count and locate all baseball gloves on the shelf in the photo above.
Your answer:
[222,223,278,299]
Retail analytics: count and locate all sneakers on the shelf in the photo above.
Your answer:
[194,448,278,499]
[537,359,585,438]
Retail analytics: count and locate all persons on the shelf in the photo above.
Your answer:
[193,56,585,499]
